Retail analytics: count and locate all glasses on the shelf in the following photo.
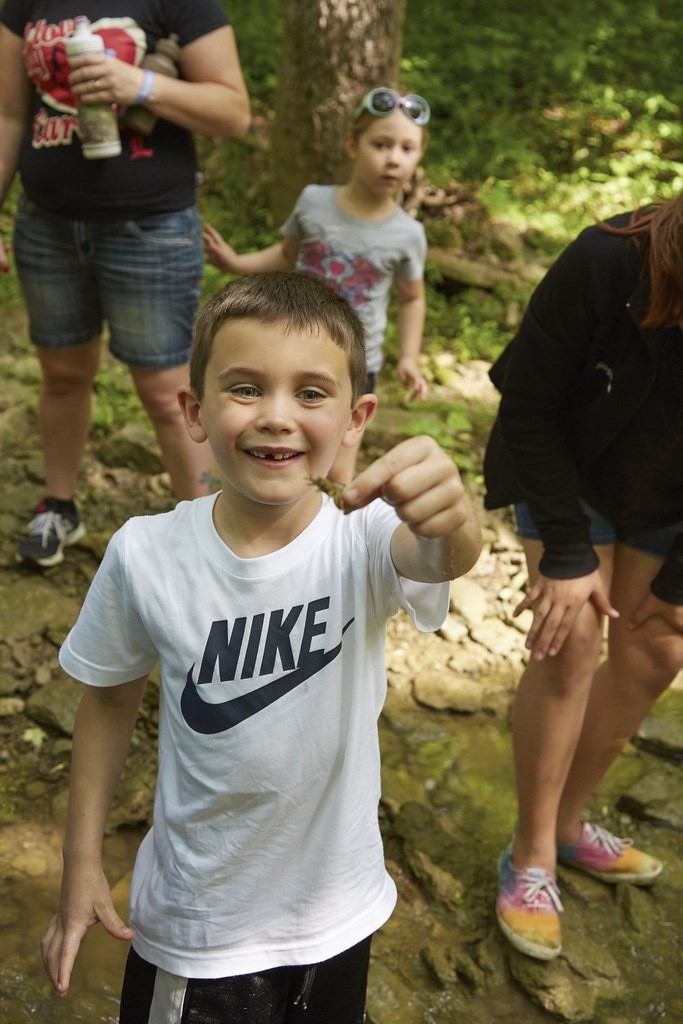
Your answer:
[354,87,431,126]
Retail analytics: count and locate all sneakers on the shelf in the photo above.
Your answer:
[558,819,664,886]
[15,497,85,568]
[496,840,564,962]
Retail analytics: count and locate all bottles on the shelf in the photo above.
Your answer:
[63,16,123,160]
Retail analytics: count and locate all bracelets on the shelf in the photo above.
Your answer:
[133,70,155,106]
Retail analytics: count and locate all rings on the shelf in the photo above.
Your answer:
[94,79,100,90]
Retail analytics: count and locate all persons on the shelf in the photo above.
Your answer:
[481,187,683,960]
[41,268,481,1024]
[0,1,252,567]
[201,87,431,484]
